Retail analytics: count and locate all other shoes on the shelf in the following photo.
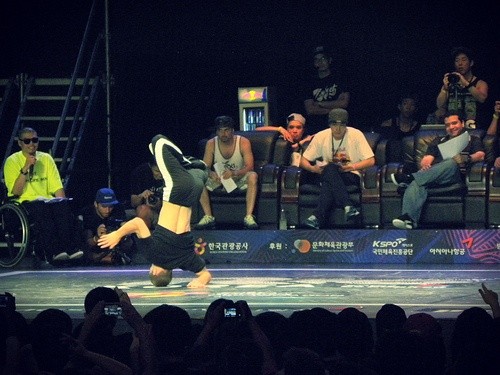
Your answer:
[55,253,70,261]
[304,219,319,229]
[392,217,414,229]
[71,250,84,259]
[344,210,359,222]
[391,173,412,186]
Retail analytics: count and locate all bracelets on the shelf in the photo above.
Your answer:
[21,168,28,175]
[275,126,282,132]
[297,141,301,147]
[464,154,472,164]
[441,89,448,95]
[493,114,499,119]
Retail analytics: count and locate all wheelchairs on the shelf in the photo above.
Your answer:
[0,177,87,270]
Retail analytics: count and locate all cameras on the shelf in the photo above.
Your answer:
[103,303,122,316]
[148,191,161,205]
[0,295,8,306]
[448,73,460,83]
[223,307,240,318]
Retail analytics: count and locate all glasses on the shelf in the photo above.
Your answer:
[18,137,39,144]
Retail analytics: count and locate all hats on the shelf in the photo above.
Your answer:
[328,109,348,125]
[286,113,306,126]
[96,189,120,205]
[312,45,330,57]
[215,116,234,129]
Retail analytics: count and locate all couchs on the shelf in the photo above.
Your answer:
[280,128,391,226]
[381,125,497,226]
[488,165,500,229]
[195,128,291,229]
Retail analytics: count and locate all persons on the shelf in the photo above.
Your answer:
[130,163,166,231]
[391,109,485,229]
[4,127,83,260]
[486,92,500,187]
[378,94,421,139]
[254,113,313,156]
[0,283,500,375]
[84,188,136,265]
[299,108,375,229]
[426,108,444,124]
[436,49,488,128]
[302,45,351,133]
[97,134,212,287]
[197,116,259,227]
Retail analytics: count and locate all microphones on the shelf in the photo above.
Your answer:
[29,151,35,180]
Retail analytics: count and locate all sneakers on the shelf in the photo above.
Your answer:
[244,216,258,229]
[195,216,215,229]
